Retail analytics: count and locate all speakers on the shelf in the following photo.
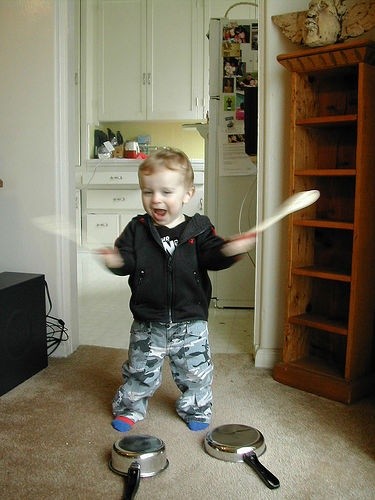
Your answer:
[0,272,48,396]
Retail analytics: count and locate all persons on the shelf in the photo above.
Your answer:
[90,147,258,431]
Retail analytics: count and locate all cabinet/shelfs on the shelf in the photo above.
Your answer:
[271,39,375,405]
[80,0,209,122]
[81,159,205,348]
[0,271,48,397]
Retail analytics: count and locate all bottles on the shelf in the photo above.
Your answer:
[98,144,111,160]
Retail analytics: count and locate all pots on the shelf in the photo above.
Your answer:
[107,432,170,500]
[203,423,281,490]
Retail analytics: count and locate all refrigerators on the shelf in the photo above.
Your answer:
[203,16,260,309]
